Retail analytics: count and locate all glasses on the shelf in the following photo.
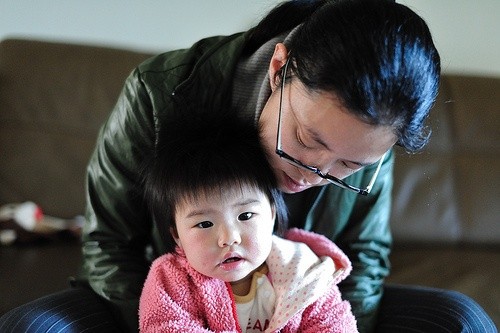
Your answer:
[274,57,388,196]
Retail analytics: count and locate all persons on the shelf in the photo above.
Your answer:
[137,114,359,333]
[0,1,499,333]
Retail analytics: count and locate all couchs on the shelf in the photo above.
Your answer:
[0,40,500,333]
